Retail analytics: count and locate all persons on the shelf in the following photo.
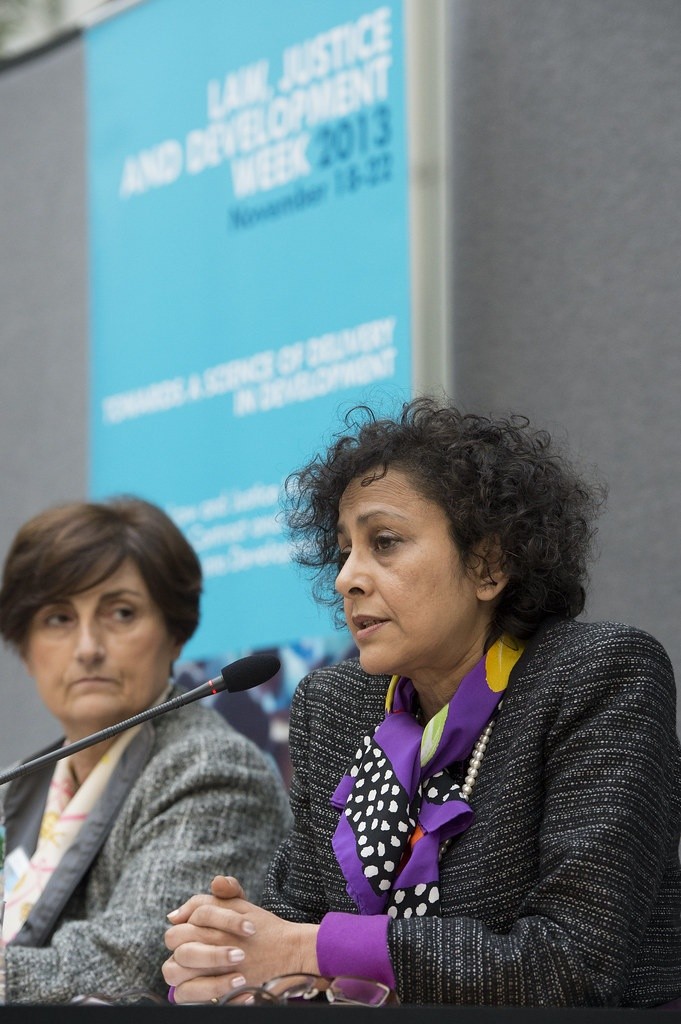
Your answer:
[161,398,681,1013]
[0,493,293,1005]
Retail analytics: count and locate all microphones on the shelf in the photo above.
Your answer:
[0,654,281,784]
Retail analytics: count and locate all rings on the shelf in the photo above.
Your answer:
[211,998,219,1004]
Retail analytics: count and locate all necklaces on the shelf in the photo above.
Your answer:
[415,708,422,720]
[436,719,495,864]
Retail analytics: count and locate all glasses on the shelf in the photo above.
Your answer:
[255,974,400,1008]
